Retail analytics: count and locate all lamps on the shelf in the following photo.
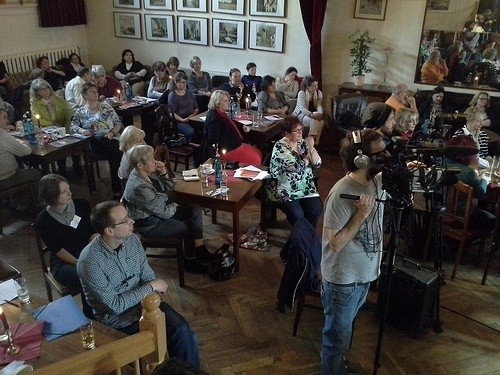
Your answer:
[375,47,394,90]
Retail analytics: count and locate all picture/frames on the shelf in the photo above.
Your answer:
[353,0,388,21]
[111,0,285,53]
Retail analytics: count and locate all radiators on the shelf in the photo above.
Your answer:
[0,46,81,75]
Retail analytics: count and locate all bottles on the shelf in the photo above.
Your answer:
[246,94,252,115]
[214,159,223,184]
[228,97,235,116]
[124,82,131,99]
[22,119,37,144]
[201,168,209,188]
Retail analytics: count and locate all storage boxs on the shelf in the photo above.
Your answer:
[0,258,22,305]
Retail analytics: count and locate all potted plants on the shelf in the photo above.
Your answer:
[348,29,376,87]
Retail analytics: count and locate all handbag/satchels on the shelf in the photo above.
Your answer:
[227,224,269,252]
[209,243,236,281]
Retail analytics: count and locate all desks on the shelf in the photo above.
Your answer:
[228,108,287,145]
[172,158,271,264]
[338,81,395,103]
[0,292,132,373]
[8,126,94,184]
[108,95,160,134]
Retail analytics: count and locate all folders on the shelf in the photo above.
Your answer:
[33,294,90,342]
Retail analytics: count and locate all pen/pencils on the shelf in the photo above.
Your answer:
[4,300,19,307]
[210,188,216,196]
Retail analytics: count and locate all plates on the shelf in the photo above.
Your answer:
[208,168,214,174]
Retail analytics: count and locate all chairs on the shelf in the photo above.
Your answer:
[0,66,500,375]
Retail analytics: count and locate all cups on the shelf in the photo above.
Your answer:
[251,111,263,128]
[14,277,31,304]
[58,127,66,137]
[203,164,212,173]
[79,319,95,349]
[219,181,227,196]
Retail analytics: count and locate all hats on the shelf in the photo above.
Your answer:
[464,21,473,28]
[361,102,392,129]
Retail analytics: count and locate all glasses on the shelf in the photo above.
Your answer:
[478,98,488,102]
[104,212,130,228]
[368,148,386,157]
[292,128,302,133]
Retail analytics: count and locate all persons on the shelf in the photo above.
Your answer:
[421,19,500,92]
[0,49,500,375]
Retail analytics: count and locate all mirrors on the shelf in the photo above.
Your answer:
[414,0,500,93]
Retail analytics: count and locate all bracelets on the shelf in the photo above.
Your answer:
[109,130,115,136]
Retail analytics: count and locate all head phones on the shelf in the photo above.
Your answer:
[352,129,370,169]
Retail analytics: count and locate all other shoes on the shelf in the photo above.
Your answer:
[196,252,215,263]
[183,259,209,274]
[344,358,361,375]
